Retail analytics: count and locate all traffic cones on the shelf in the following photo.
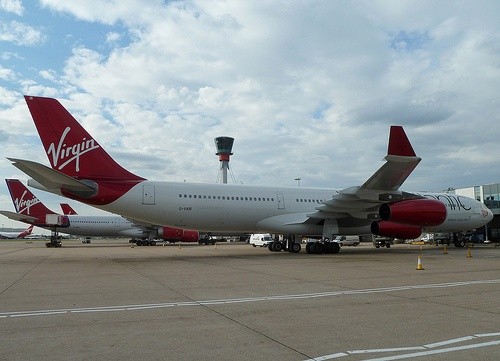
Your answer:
[441,245,448,254]
[465,249,474,258]
[416,256,426,270]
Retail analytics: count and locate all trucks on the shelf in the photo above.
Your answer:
[371,233,390,248]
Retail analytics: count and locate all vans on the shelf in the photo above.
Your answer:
[249,234,275,247]
[420,234,435,243]
[332,235,360,247]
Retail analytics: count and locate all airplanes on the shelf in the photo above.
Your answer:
[5,95,494,255]
[0,178,255,245]
[0,224,35,240]
